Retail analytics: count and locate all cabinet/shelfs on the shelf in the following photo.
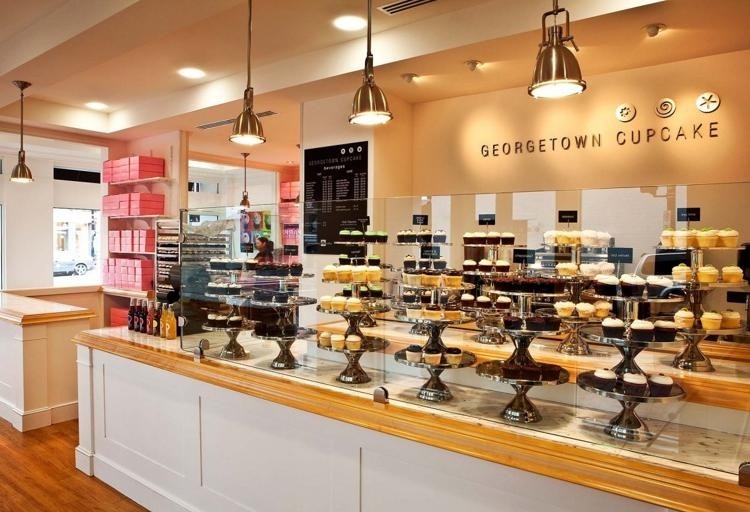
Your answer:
[101,176,172,300]
[154,217,231,312]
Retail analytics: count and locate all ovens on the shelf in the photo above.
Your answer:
[201,234,742,407]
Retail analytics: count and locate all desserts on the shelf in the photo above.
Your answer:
[319,226,744,367]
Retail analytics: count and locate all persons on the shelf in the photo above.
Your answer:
[254,236,274,263]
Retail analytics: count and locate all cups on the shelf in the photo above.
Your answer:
[243,224,271,229]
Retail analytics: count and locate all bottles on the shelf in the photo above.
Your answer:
[127,297,177,340]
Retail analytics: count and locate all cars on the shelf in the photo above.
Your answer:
[52,250,94,276]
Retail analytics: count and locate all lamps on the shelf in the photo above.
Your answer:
[229,0,267,147]
[345,0,393,127]
[527,0,587,99]
[9,80,33,183]
[240,152,251,210]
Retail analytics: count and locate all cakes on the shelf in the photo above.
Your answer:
[204,257,303,336]
